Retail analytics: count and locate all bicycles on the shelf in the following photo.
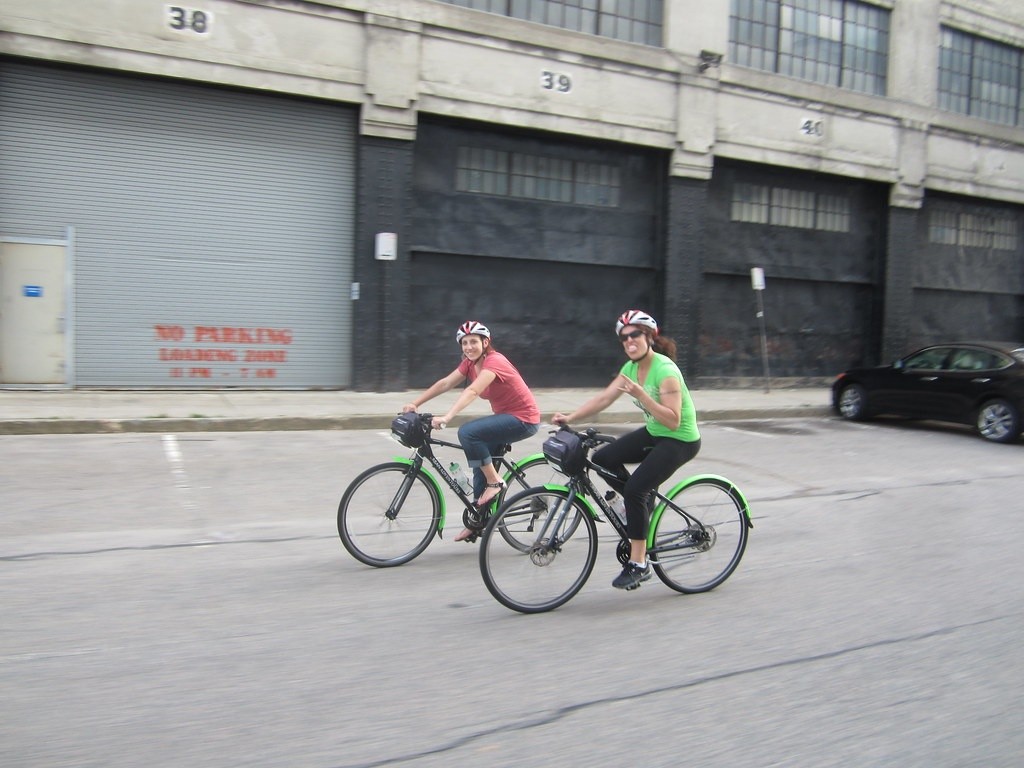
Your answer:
[336,408,591,568]
[477,419,754,614]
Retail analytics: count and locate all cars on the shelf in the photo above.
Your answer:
[829,339,1023,444]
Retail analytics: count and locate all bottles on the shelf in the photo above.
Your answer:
[450,462,472,494]
[605,490,627,525]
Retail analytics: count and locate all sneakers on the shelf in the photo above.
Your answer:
[612,561,651,588]
[647,488,658,516]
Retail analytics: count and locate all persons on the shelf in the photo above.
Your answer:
[552,310,701,588]
[403,320,541,541]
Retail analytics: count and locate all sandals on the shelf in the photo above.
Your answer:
[476,480,507,508]
[453,528,478,542]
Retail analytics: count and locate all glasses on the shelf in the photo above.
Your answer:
[619,330,649,343]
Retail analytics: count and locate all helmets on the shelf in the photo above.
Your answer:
[616,310,658,337]
[456,321,490,343]
